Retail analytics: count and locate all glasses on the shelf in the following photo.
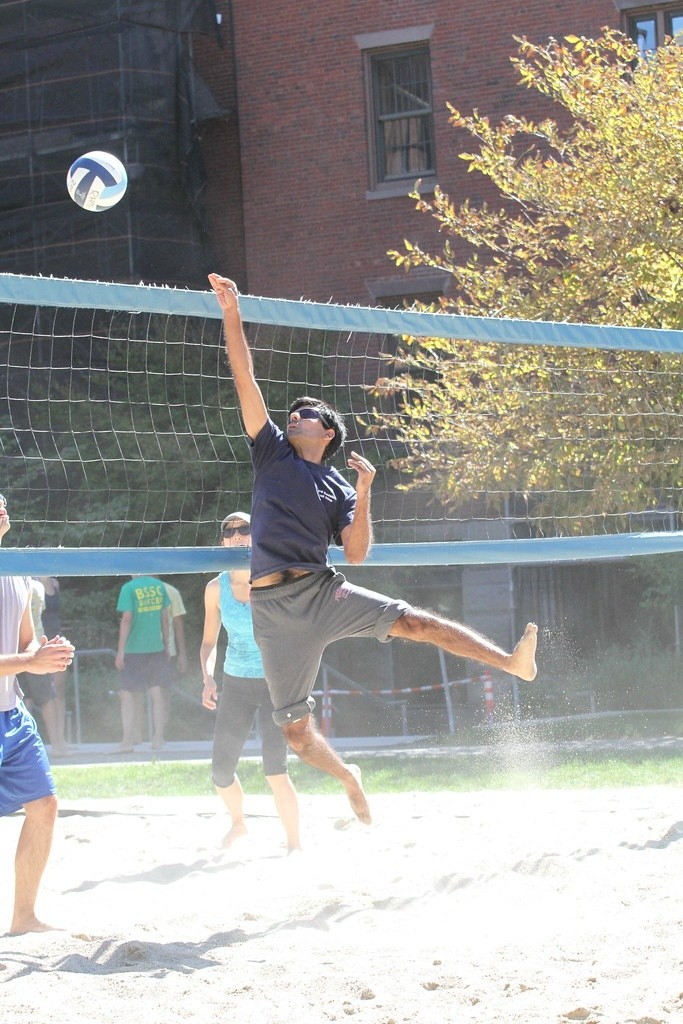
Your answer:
[289,409,331,429]
[224,525,251,538]
[0,494,7,506]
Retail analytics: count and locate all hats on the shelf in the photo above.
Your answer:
[221,511,251,536]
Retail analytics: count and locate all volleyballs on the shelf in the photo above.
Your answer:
[66,149,133,214]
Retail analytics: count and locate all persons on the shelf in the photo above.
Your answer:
[208,269,540,825]
[108,576,191,758]
[0,489,89,944]
[27,536,76,757]
[199,510,302,853]
